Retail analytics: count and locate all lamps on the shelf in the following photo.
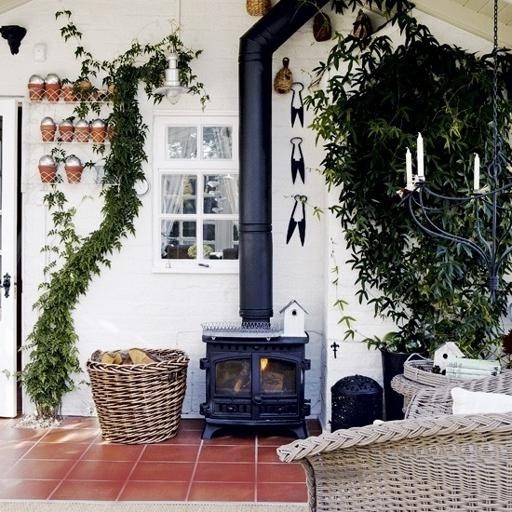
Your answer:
[154,25,192,103]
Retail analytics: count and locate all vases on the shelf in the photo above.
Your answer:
[29,84,118,185]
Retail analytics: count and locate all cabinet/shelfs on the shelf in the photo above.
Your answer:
[27,84,118,193]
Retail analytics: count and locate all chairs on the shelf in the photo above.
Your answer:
[271,371,511,512]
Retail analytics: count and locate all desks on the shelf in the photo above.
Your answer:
[391,354,511,413]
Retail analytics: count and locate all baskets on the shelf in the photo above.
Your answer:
[87,348,190,446]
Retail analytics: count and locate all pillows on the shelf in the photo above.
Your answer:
[448,386,511,415]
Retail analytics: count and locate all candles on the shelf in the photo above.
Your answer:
[417,134,425,178]
[405,147,413,183]
[474,150,479,188]
